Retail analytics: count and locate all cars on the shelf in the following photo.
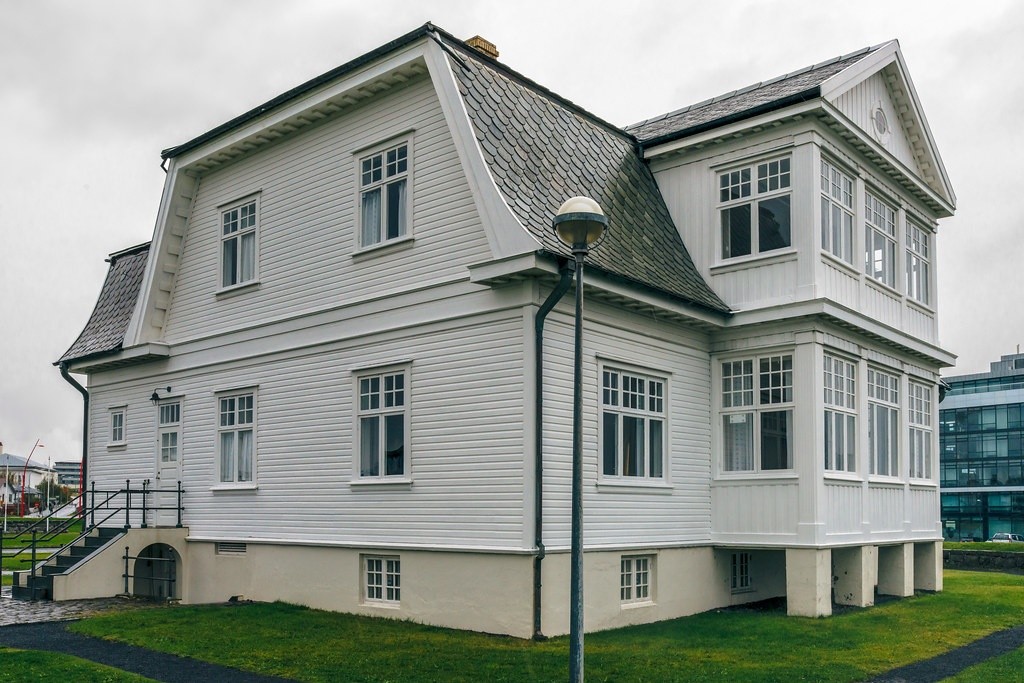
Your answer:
[986,533,1024,544]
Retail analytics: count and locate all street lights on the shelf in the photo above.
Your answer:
[20,438,45,517]
[549,195,610,683]
